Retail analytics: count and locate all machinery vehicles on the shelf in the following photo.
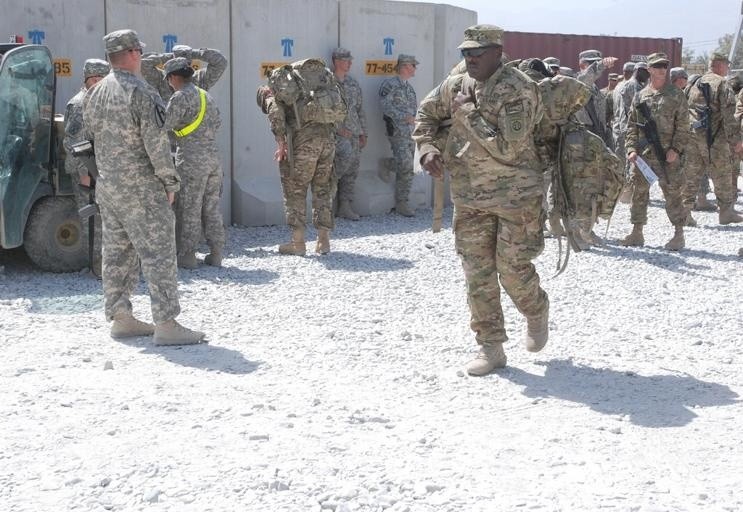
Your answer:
[0,40,91,275]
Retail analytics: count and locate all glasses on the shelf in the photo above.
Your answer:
[407,64,417,67]
[461,48,499,57]
[653,63,669,69]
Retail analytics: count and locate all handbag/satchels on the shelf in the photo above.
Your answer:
[533,75,593,145]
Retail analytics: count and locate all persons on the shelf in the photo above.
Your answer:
[378,54,419,217]
[256,84,335,255]
[411,25,549,376]
[330,48,368,221]
[82,29,205,345]
[162,57,226,268]
[63,58,110,279]
[141,44,228,105]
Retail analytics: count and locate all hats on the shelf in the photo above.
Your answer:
[647,52,671,66]
[577,49,600,62]
[83,57,111,82]
[331,47,354,62]
[161,57,189,80]
[172,44,194,63]
[687,74,701,82]
[456,24,506,51]
[256,84,270,115]
[102,28,146,57]
[670,67,688,81]
[558,66,575,79]
[397,52,420,66]
[607,61,648,82]
[541,55,563,71]
[710,51,732,65]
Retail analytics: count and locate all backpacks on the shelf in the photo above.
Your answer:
[269,57,352,127]
[552,123,627,233]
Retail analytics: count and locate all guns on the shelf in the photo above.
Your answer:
[692,83,711,163]
[383,114,394,137]
[71,140,98,218]
[583,97,609,147]
[635,101,669,184]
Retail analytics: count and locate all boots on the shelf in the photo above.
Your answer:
[679,187,743,227]
[110,305,156,339]
[525,294,550,353]
[278,225,308,256]
[204,245,223,268]
[620,186,635,204]
[543,212,564,239]
[395,200,414,218]
[335,195,360,221]
[467,340,508,378]
[153,319,208,346]
[579,228,606,246]
[665,225,685,250]
[315,226,331,255]
[175,250,201,269]
[378,157,392,184]
[619,224,646,247]
